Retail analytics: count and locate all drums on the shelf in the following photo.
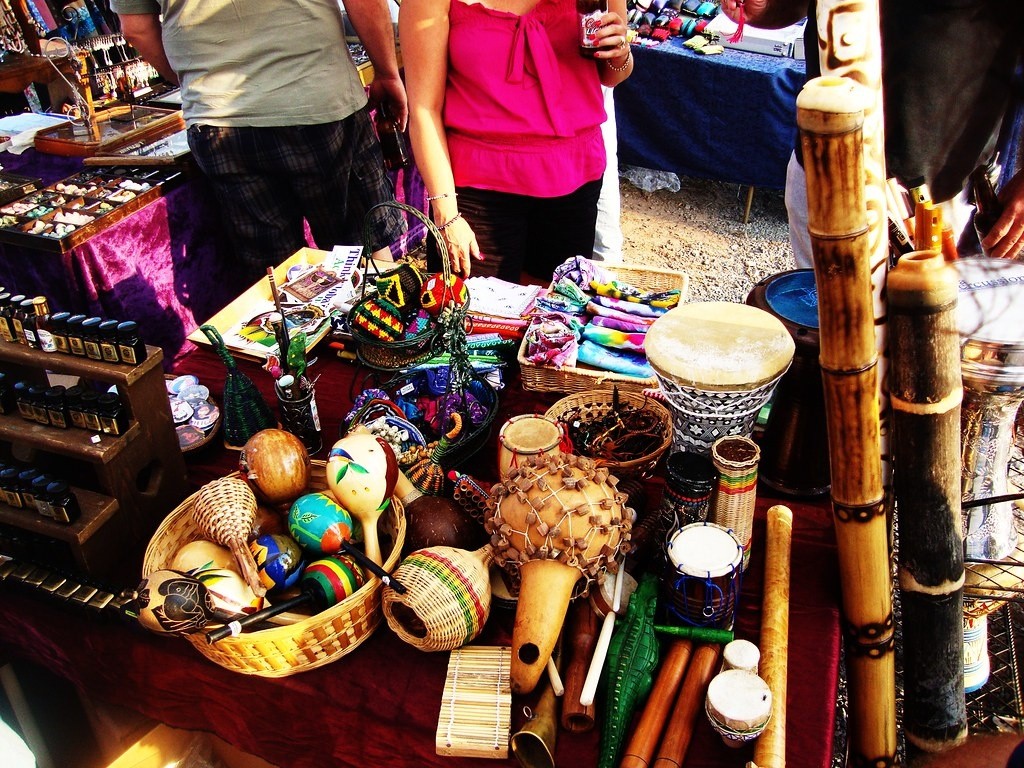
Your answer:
[750,264,830,487]
[945,254,1022,565]
[645,299,798,458]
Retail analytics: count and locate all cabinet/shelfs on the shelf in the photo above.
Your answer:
[0,346,188,571]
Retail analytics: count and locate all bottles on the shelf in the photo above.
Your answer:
[576,0,607,60]
[0,286,148,525]
[376,102,409,169]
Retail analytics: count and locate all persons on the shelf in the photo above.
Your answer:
[109,0,408,298]
[721,0,1024,267]
[398,0,633,290]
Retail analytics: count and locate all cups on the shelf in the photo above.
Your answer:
[655,451,716,562]
[276,386,321,457]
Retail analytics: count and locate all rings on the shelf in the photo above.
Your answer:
[459,256,470,262]
[617,36,625,48]
[449,259,460,264]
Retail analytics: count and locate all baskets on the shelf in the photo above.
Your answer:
[142,460,407,678]
[518,259,688,394]
[346,201,470,367]
[544,389,672,483]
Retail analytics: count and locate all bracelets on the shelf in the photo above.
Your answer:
[437,212,462,230]
[425,193,458,200]
[606,43,631,72]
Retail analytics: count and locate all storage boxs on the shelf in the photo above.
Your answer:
[704,13,809,57]
[0,160,192,254]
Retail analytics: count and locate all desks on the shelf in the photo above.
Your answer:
[0,44,429,368]
[569,14,808,223]
[1,351,842,768]
